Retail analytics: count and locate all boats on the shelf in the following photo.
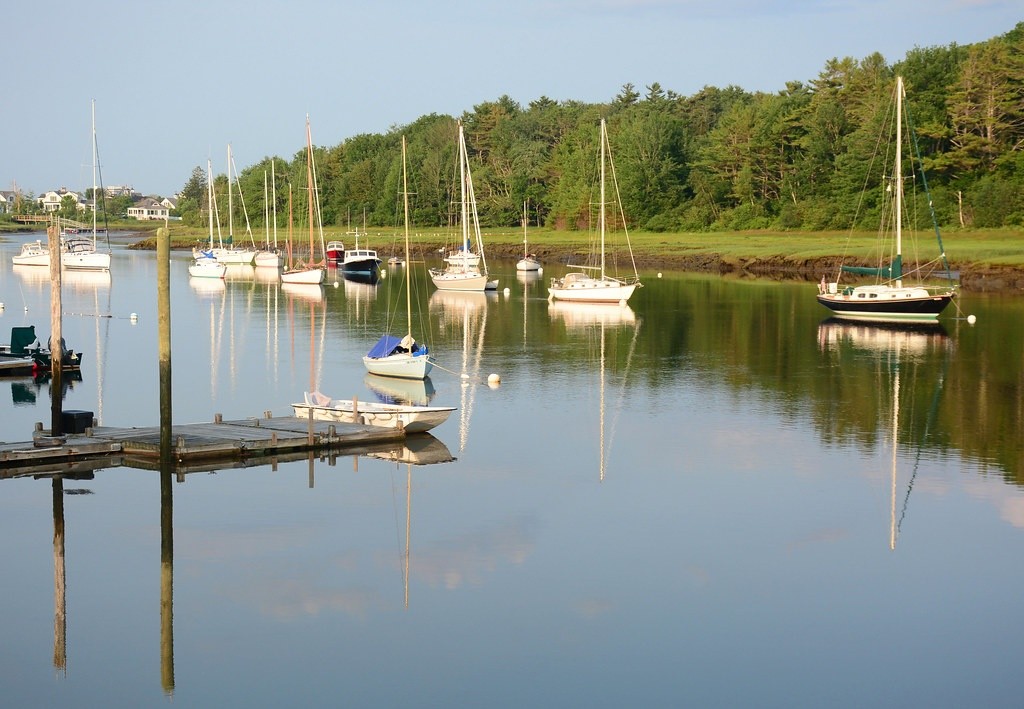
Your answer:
[290,389,466,437]
[344,274,382,303]
[362,429,458,469]
[0,325,86,377]
[323,239,347,264]
[340,228,385,281]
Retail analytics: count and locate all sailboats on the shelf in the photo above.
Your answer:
[190,145,285,267]
[12,99,114,273]
[829,81,958,326]
[190,276,229,392]
[188,160,228,279]
[518,199,544,270]
[547,299,643,482]
[277,113,328,285]
[429,126,502,295]
[367,135,440,380]
[363,373,436,409]
[547,117,644,304]
[515,270,542,350]
